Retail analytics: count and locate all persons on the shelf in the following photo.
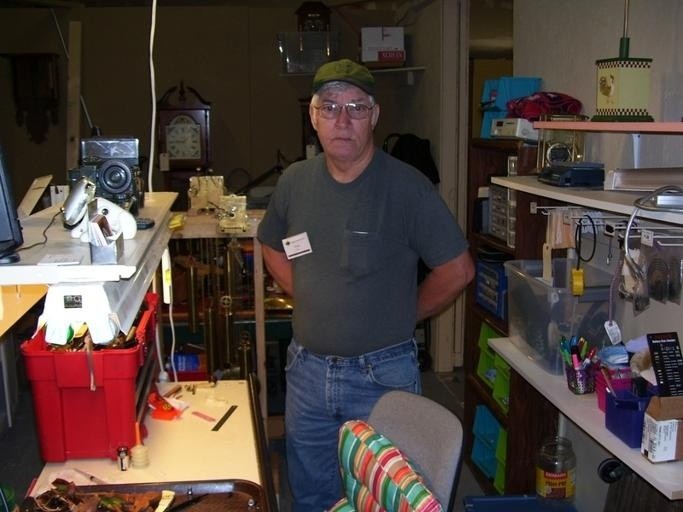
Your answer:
[255,60,477,512]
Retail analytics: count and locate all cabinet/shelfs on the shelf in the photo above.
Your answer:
[487,121,683,501]
[464,138,567,497]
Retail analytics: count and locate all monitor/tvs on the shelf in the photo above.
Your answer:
[0,150,24,264]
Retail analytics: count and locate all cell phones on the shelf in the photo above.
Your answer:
[135,218,154,229]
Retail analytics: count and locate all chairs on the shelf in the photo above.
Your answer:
[367,390,463,512]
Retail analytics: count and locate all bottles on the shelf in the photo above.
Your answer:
[117,446,129,471]
[533,436,576,503]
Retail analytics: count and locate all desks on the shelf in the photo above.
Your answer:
[28,380,269,512]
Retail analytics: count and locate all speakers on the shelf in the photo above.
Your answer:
[80,135,140,218]
[69,165,97,195]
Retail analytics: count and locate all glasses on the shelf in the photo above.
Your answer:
[311,103,374,119]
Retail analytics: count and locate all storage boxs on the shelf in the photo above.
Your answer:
[361,25,407,69]
[503,258,617,374]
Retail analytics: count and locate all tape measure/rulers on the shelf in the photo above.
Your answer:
[212,406,237,431]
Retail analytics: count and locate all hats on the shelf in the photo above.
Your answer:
[312,58,376,95]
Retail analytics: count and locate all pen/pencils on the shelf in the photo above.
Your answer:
[559,336,596,394]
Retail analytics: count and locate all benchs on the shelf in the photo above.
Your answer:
[163,287,292,417]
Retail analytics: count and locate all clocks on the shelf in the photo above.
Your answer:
[155,80,213,254]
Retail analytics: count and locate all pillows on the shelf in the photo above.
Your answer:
[326,419,444,512]
